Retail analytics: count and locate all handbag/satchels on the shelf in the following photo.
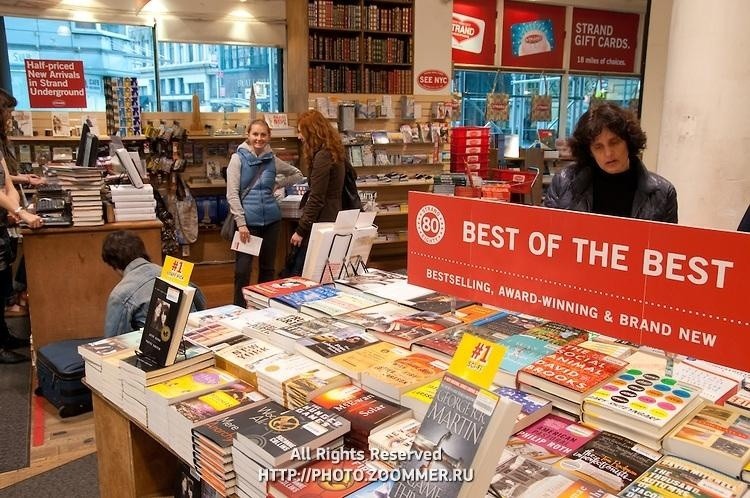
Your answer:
[220,211,236,240]
[154,188,199,255]
[342,171,366,212]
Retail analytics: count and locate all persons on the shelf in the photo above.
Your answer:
[537,101,686,227]
[0,88,47,365]
[100,231,209,339]
[223,120,303,312]
[288,109,348,272]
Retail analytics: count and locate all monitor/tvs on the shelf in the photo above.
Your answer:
[83,133,98,167]
[75,124,90,166]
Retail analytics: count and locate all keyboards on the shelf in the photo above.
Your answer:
[36,199,65,213]
[38,184,62,192]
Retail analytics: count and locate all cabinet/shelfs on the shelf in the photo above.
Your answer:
[308,94,450,245]
[308,0,413,118]
[0,109,303,233]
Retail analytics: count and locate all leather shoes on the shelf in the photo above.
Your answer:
[7,334,29,349]
[1,348,28,363]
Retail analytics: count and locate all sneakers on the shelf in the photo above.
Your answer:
[4,306,28,319]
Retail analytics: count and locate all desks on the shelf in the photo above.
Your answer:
[19,196,164,385]
[80,375,239,498]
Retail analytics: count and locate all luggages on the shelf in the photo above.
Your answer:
[35,338,105,417]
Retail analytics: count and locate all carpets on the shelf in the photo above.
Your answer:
[0,316,32,473]
[0,447,98,498]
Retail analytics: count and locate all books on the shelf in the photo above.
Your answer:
[305,220,378,284]
[76,273,745,497]
[44,160,163,227]
[278,193,304,220]
[181,156,229,188]
[310,0,458,250]
[227,229,264,260]
